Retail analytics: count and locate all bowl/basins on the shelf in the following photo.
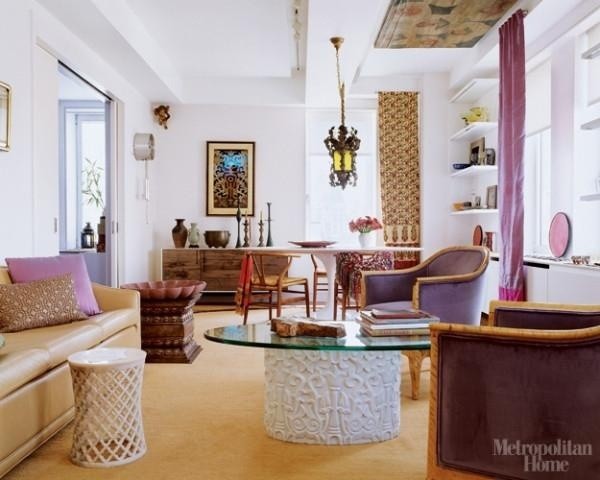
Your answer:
[205,231,230,248]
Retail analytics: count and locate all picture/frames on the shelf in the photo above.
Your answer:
[0,80,13,153]
[469,137,484,166]
[486,184,498,210]
[205,140,256,218]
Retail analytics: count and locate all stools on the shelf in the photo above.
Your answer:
[64,347,150,470]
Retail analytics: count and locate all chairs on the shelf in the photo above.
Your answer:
[310,247,360,313]
[332,250,396,321]
[358,243,491,403]
[427,300,599,479]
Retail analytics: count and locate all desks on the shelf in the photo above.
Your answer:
[240,244,426,321]
[60,247,106,285]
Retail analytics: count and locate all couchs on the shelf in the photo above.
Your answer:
[1,263,143,477]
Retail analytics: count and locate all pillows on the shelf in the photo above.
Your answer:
[5,253,105,316]
[1,272,89,333]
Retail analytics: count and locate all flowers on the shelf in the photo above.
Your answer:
[348,215,383,235]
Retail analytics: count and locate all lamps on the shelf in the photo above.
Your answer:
[322,33,361,187]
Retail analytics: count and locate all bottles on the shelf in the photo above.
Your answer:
[486,231,493,252]
[96,216,105,251]
[479,148,495,165]
[187,222,202,248]
[172,218,187,248]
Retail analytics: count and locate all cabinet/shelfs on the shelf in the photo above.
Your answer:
[578,37,599,205]
[161,247,290,305]
[446,76,499,218]
[481,252,600,314]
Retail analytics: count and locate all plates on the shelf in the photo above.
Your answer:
[453,164,469,169]
[548,211,571,259]
[472,225,482,247]
[288,240,336,247]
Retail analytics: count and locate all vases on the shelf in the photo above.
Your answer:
[358,230,377,250]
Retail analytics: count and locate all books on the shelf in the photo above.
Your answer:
[359,310,441,323]
[372,306,424,319]
[356,316,429,329]
[359,329,431,337]
